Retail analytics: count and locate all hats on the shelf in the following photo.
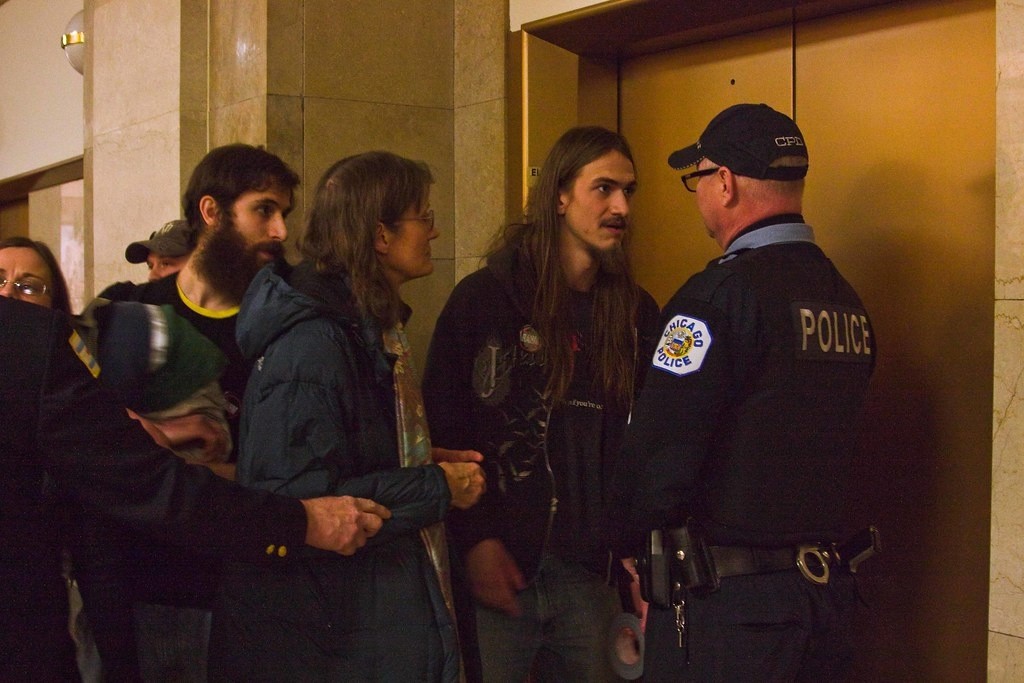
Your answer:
[125,220,197,264]
[668,102,809,181]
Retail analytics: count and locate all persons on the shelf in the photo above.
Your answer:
[218,152,486,683]
[614,101,877,681]
[0,236,109,683]
[77,138,302,683]
[423,125,663,683]
[0,294,392,683]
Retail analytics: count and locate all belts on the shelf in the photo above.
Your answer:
[710,545,835,576]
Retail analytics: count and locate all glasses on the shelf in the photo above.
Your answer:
[395,210,434,230]
[681,168,720,192]
[0,275,56,298]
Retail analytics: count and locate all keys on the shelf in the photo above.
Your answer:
[670,600,686,649]
